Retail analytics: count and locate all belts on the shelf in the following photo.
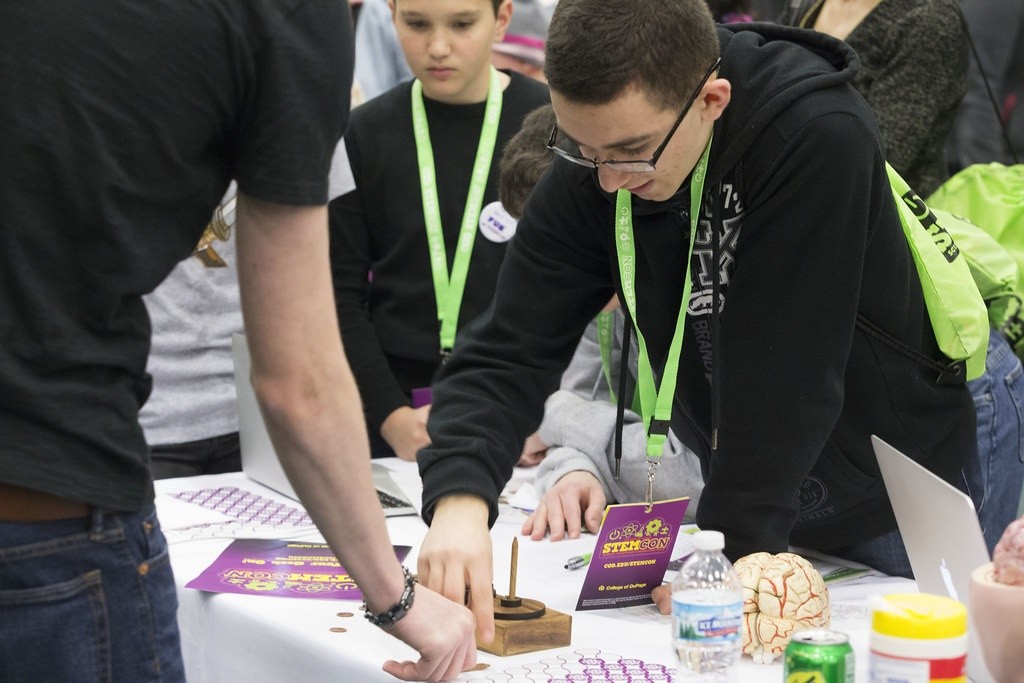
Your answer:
[0,486,119,523]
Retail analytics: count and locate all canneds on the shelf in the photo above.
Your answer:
[783,628,856,683]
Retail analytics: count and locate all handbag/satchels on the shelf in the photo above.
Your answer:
[929,206,1024,365]
[924,161,1024,274]
[880,151,990,381]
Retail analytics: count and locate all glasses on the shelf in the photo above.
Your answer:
[546,57,721,172]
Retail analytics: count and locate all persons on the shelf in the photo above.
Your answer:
[136,0,1024,585]
[415,1,988,647]
[0,0,477,683]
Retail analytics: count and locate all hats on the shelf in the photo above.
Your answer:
[491,0,547,66]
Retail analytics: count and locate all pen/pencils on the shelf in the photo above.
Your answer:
[564,550,594,571]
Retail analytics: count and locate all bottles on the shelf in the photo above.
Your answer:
[866,590,968,683]
[669,530,745,683]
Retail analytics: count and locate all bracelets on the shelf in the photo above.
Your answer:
[364,560,418,627]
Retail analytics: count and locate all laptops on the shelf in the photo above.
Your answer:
[872,434,994,683]
[232,332,415,516]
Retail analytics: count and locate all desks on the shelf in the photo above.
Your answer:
[155,459,896,683]
